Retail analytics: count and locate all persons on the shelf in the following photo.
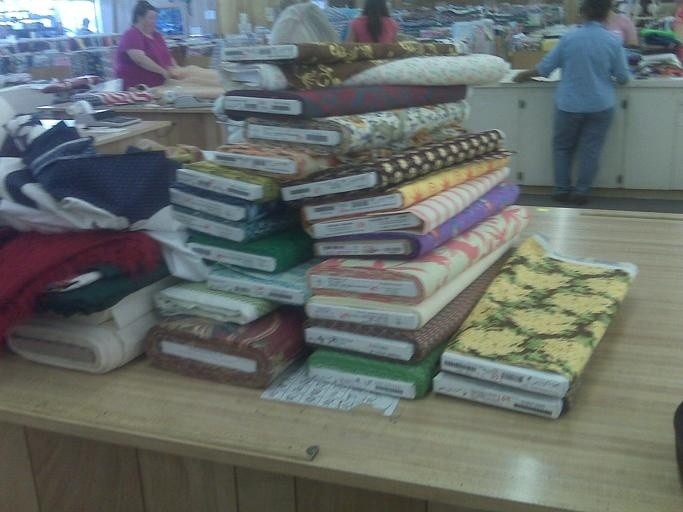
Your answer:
[111,1,177,92]
[344,1,398,44]
[602,7,639,48]
[73,18,93,35]
[511,1,630,206]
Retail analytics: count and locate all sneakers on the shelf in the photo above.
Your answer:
[574,196,587,205]
[552,193,568,204]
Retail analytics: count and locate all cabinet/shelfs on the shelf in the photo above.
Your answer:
[474,76,683,196]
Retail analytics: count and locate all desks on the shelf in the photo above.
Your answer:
[0,208,683,509]
